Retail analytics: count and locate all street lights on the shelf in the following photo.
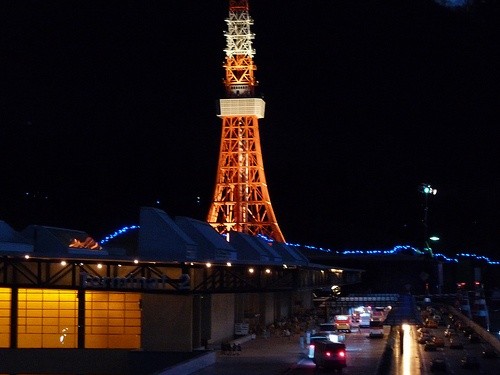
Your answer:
[420,183,440,299]
[0,255,30,374]
[60,260,103,375]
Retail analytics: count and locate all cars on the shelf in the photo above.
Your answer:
[481,349,497,361]
[430,357,447,374]
[413,279,484,351]
[460,356,480,369]
[298,302,391,374]
